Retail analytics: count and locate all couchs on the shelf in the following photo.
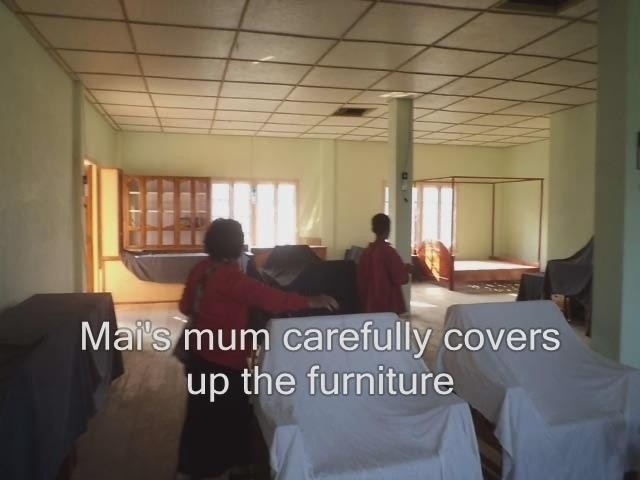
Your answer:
[254,311,482,480]
[440,300,640,479]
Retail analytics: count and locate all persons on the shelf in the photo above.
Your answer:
[172,219,340,480]
[356,213,409,317]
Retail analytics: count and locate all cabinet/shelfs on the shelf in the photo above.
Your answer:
[119,176,212,251]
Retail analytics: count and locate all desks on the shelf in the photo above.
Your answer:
[1,292,124,479]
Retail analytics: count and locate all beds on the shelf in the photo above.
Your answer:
[414,175,546,291]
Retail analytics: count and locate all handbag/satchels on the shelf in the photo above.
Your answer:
[173,262,225,364]
